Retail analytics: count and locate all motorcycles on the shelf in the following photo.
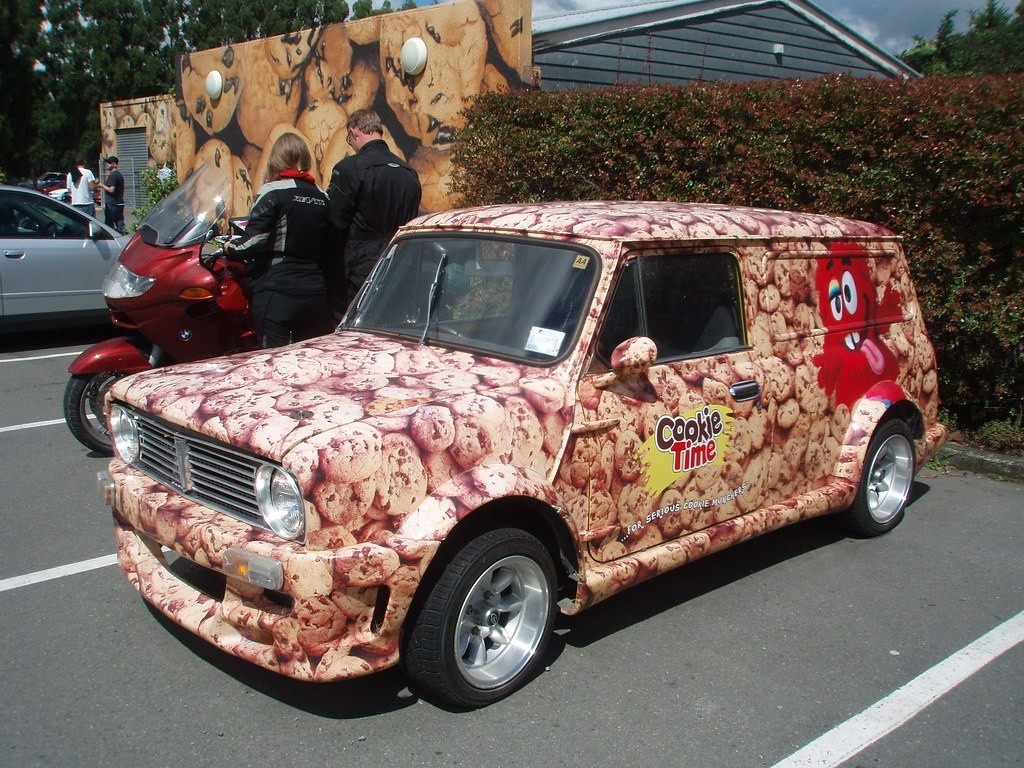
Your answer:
[62,161,264,456]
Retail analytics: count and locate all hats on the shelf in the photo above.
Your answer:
[105,156,118,164]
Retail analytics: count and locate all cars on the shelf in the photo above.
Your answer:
[36,172,100,206]
[100,192,950,714]
[0,185,137,330]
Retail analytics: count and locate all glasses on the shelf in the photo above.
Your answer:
[107,161,111,164]
[346,124,358,145]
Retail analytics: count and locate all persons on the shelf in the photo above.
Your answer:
[221,131,345,350]
[96,156,125,233]
[66,155,98,217]
[324,108,423,328]
